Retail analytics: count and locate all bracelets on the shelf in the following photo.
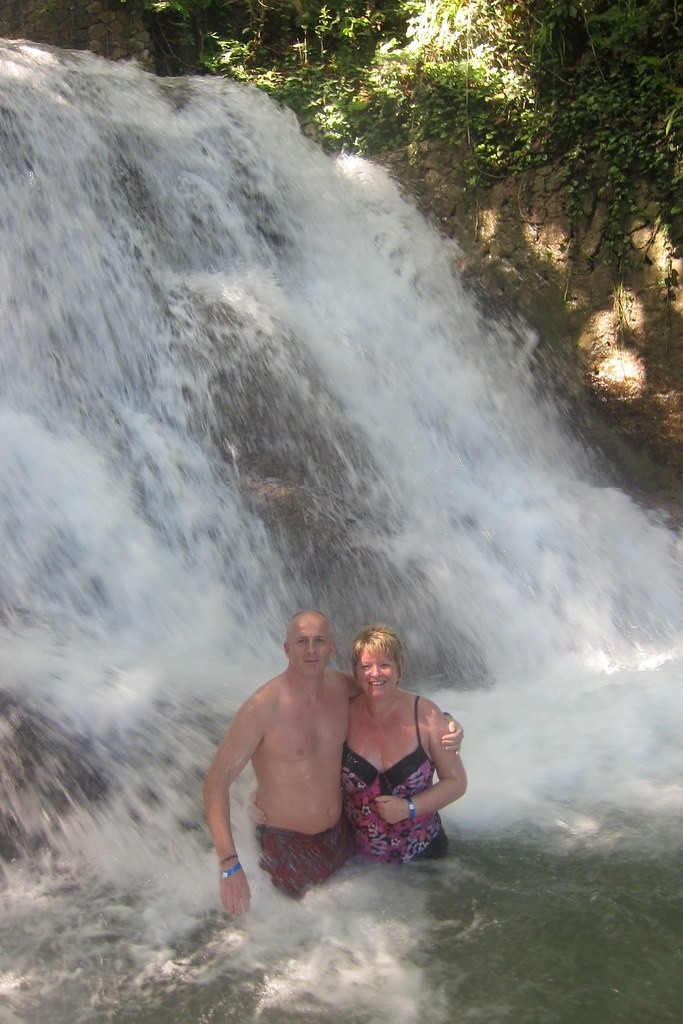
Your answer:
[406,797,416,819]
[218,854,238,865]
[442,711,453,719]
[217,861,241,877]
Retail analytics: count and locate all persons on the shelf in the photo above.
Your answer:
[247,623,469,865]
[201,609,463,918]
